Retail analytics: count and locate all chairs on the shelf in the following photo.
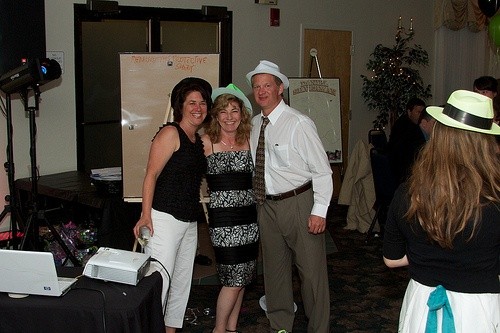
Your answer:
[338,129,389,247]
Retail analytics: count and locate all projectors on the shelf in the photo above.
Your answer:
[83,247,151,286]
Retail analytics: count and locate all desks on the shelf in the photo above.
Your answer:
[14,166,145,255]
[0,266,167,333]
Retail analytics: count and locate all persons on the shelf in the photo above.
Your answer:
[246,59,333,333]
[471,76,500,127]
[200,84,260,333]
[133,77,213,333]
[384,97,437,192]
[384,89,500,333]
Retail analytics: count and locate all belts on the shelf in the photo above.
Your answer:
[267,182,312,201]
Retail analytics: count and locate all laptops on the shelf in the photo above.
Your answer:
[0,249,78,296]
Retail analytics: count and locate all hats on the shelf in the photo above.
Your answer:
[212,83,253,118]
[246,60,289,91]
[425,90,500,135]
[170,78,215,110]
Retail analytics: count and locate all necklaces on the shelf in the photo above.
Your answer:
[221,140,233,149]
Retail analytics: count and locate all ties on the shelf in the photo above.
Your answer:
[255,118,271,206]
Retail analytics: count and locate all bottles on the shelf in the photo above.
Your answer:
[137,225,151,248]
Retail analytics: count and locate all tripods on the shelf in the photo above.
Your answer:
[0,86,84,275]
